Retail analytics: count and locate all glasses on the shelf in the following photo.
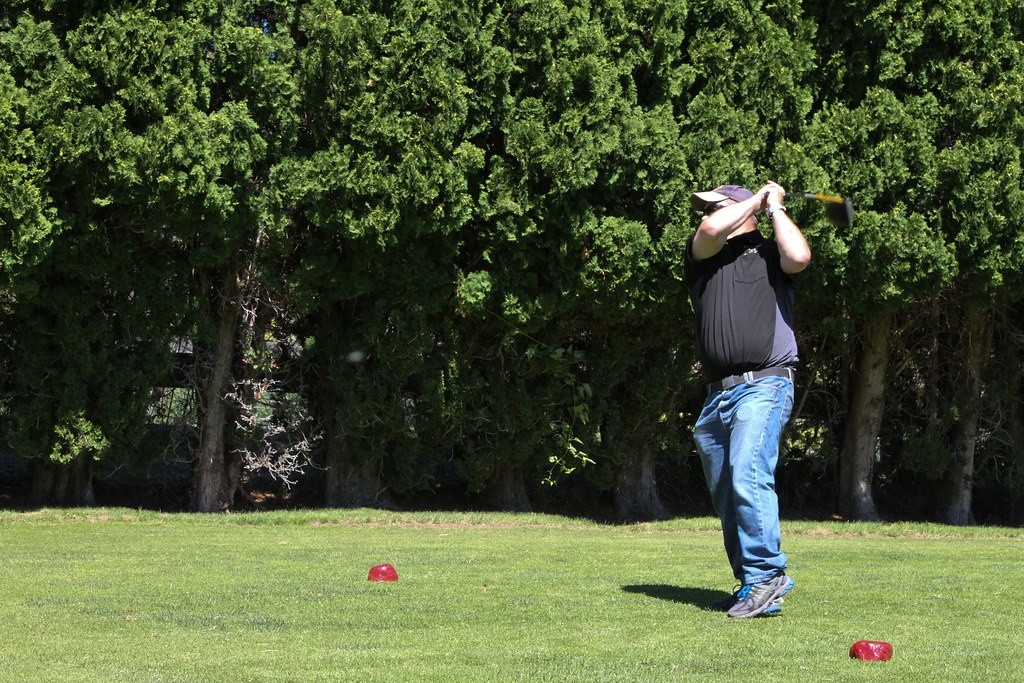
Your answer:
[702,204,727,215]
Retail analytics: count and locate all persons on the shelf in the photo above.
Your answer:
[684,179,811,617]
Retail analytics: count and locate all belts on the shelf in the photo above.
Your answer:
[705,367,795,394]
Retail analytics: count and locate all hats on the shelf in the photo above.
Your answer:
[690,185,754,211]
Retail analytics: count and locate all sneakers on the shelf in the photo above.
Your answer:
[727,570,794,618]
[719,584,784,614]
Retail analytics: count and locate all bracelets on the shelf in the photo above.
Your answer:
[766,206,786,219]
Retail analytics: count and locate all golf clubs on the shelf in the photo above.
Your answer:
[763,191,854,227]
